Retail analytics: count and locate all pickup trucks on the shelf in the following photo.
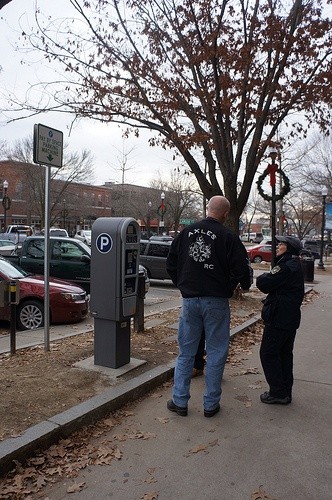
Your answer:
[0,236,149,298]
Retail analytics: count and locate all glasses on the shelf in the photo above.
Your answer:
[277,242,287,246]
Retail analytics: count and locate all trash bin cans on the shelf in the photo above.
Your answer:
[300,252,315,283]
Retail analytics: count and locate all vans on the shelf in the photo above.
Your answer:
[40,228,69,238]
[240,232,263,243]
[5,224,33,242]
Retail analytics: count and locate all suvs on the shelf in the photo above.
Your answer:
[138,235,172,280]
[301,240,321,256]
[258,237,321,261]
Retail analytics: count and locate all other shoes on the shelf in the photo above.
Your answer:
[204,403,220,418]
[167,399,188,416]
[260,390,292,405]
[190,368,204,377]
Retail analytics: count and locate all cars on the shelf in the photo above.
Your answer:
[0,254,88,330]
[74,230,91,246]
[245,244,272,263]
[0,240,22,256]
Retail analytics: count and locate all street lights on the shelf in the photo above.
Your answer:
[148,201,152,236]
[63,198,66,228]
[2,180,10,232]
[268,144,277,266]
[160,192,165,235]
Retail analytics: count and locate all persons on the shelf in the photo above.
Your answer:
[166,195,253,417]
[256,235,305,403]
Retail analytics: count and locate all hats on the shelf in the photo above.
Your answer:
[274,234,302,249]
